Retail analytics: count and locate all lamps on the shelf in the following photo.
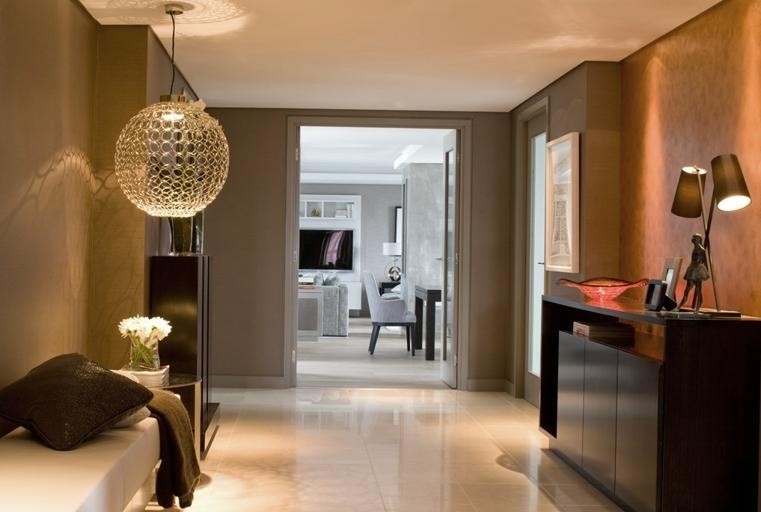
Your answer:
[383,242,402,282]
[113,4,230,218]
[670,153,752,318]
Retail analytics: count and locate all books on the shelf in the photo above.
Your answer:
[573,321,635,339]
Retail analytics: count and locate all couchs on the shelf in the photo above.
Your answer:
[298,282,349,337]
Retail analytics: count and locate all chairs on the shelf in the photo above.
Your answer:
[363,271,417,356]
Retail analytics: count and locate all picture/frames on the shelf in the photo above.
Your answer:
[644,257,682,311]
[545,132,580,274]
[394,206,403,243]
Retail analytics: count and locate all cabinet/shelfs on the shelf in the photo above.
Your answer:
[150,254,221,452]
[299,199,356,220]
[298,288,324,341]
[538,294,761,512]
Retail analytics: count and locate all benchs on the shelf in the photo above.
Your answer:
[0,392,182,512]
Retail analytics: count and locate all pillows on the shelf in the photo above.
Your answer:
[313,271,340,286]
[0,352,153,452]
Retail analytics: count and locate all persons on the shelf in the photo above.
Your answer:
[672,233,711,312]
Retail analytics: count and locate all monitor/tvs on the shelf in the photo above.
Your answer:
[298,228,355,273]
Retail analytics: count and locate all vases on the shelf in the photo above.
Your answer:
[129,332,160,371]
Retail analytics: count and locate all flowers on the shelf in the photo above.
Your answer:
[118,314,173,370]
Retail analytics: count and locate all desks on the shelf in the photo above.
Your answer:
[138,371,203,389]
[415,284,452,360]
[379,282,402,296]
